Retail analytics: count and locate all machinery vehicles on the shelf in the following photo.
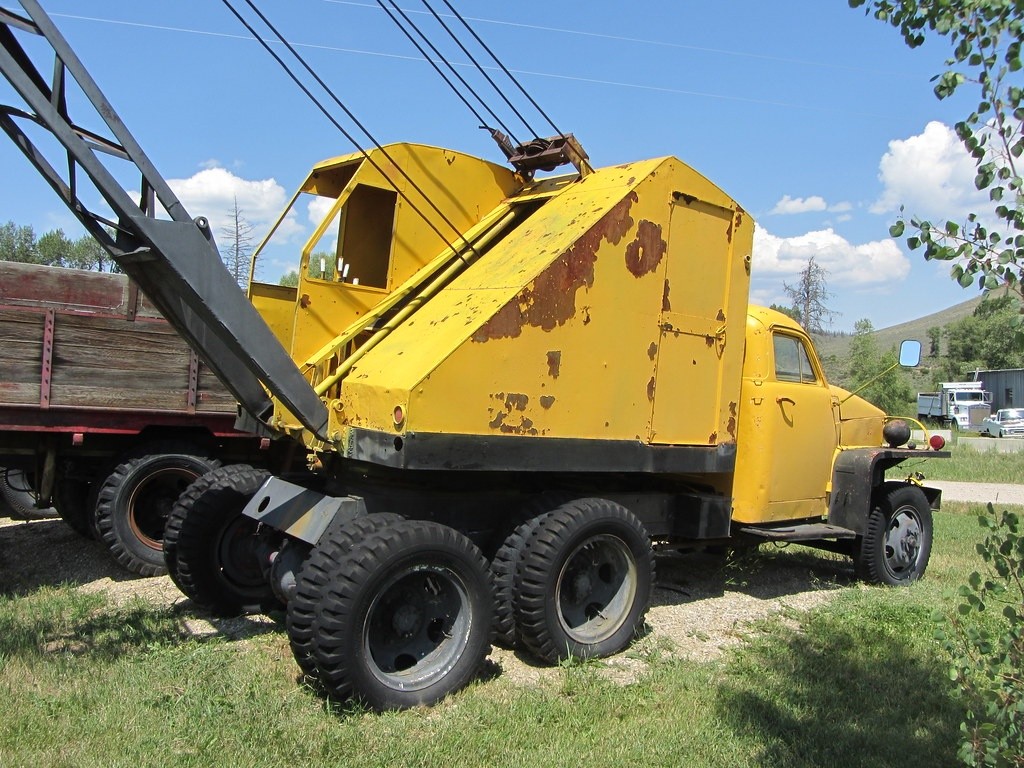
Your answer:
[0,2,954,717]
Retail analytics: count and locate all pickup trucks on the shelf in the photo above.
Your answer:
[982,408,1024,439]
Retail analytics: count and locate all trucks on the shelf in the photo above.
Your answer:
[917,381,993,434]
[0,259,281,576]
